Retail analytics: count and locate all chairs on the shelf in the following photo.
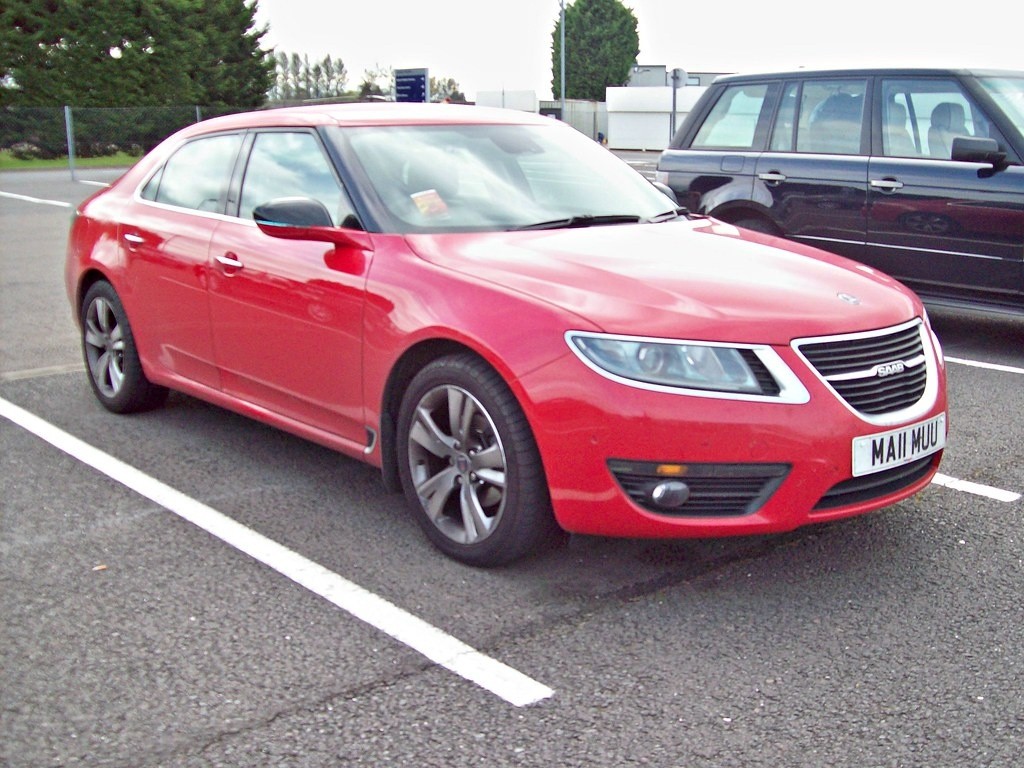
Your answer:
[887,101,971,160]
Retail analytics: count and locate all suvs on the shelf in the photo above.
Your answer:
[656,67,1024,315]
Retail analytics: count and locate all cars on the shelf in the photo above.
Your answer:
[62,100,948,573]
